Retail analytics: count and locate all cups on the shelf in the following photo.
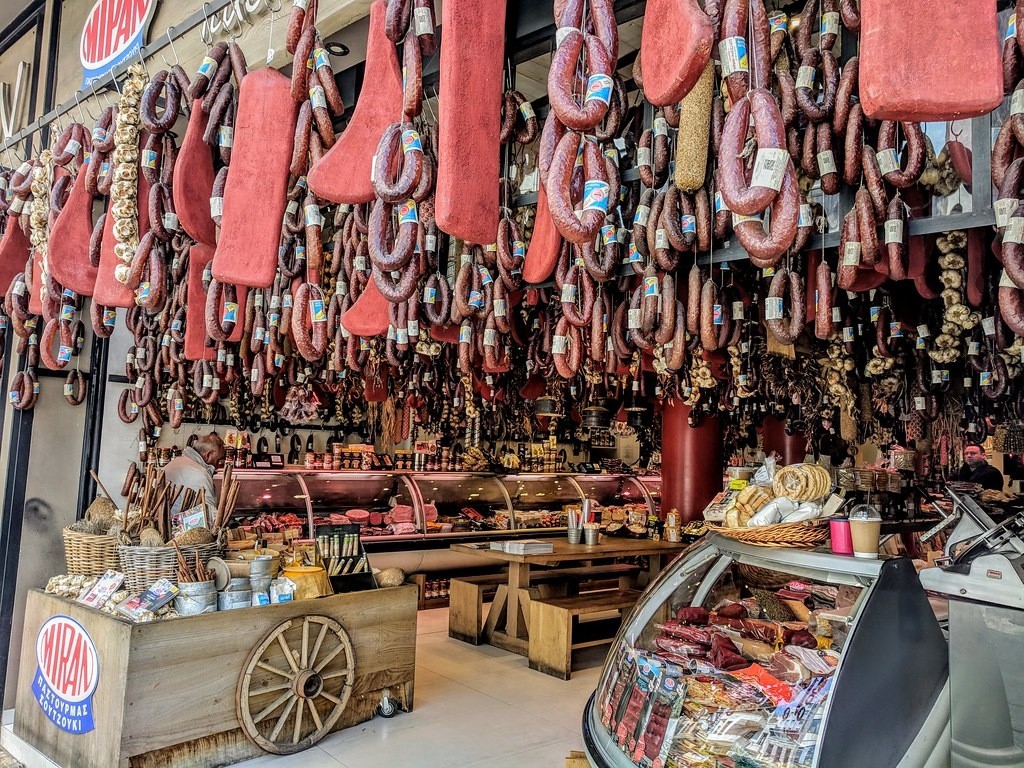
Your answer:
[567,528,581,544]
[568,498,591,529]
[828,516,853,555]
[584,528,599,545]
[848,517,883,560]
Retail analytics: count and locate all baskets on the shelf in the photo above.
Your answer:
[63,518,120,579]
[119,541,224,592]
[704,517,832,589]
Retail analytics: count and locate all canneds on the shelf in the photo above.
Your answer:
[225,448,245,461]
[427,447,462,472]
[425,580,451,600]
[519,450,562,473]
[304,443,343,471]
[146,447,181,467]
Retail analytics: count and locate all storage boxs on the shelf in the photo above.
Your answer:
[11,586,419,768]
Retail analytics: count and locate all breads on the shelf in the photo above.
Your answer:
[723,464,831,530]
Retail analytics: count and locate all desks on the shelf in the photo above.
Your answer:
[451,535,691,656]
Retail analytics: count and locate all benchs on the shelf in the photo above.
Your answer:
[528,588,666,680]
[450,564,641,648]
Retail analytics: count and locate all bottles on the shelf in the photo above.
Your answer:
[316,533,368,575]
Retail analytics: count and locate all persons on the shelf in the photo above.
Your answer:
[153,434,225,521]
[947,444,1004,492]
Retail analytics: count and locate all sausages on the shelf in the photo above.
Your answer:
[121,428,146,505]
[0,0,1024,446]
